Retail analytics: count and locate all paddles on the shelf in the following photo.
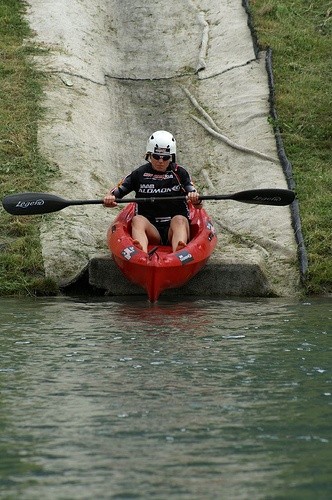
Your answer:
[2,188,295,216]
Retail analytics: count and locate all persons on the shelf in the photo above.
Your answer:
[102,130,205,257]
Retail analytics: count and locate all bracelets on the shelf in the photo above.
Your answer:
[193,199,202,206]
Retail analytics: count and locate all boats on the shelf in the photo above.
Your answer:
[106,202,217,305]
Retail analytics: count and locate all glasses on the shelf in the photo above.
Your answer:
[152,154,172,161]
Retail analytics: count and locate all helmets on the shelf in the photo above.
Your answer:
[144,130,177,163]
